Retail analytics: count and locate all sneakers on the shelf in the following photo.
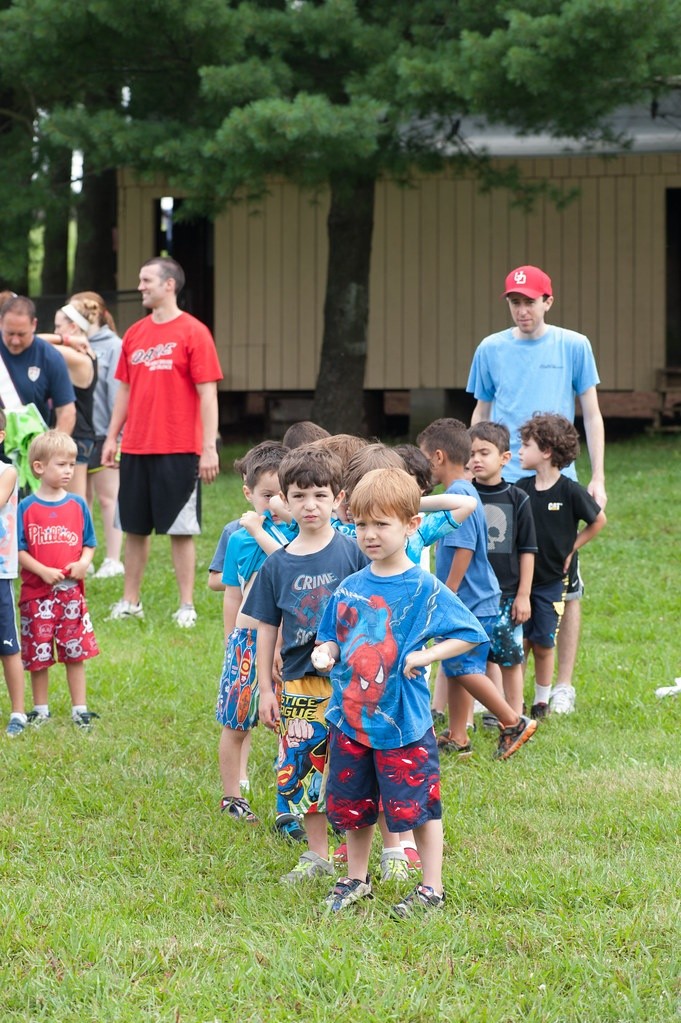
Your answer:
[473,699,488,713]
[431,708,445,729]
[493,716,537,762]
[380,852,409,887]
[334,841,348,868]
[270,814,307,847]
[402,846,424,871]
[317,872,374,917]
[389,883,446,925]
[221,797,259,828]
[86,556,125,580]
[28,710,51,731]
[482,712,500,735]
[172,607,198,627]
[328,822,346,843]
[435,729,472,761]
[103,598,144,625]
[71,710,100,736]
[465,723,477,736]
[549,685,575,716]
[281,850,336,891]
[531,702,550,720]
[6,717,27,738]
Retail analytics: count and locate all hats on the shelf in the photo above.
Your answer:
[500,265,553,301]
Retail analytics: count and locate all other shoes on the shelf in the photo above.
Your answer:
[239,780,250,795]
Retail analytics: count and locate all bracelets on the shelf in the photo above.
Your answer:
[64,334,70,346]
[59,334,63,344]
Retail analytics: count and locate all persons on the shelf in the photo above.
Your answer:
[0,291,76,499]
[38,299,99,498]
[72,291,124,580]
[99,256,224,628]
[15,430,100,732]
[416,418,538,761]
[242,444,370,884]
[207,421,479,843]
[465,265,607,713]
[468,411,609,734]
[1,410,26,736]
[312,469,491,917]
